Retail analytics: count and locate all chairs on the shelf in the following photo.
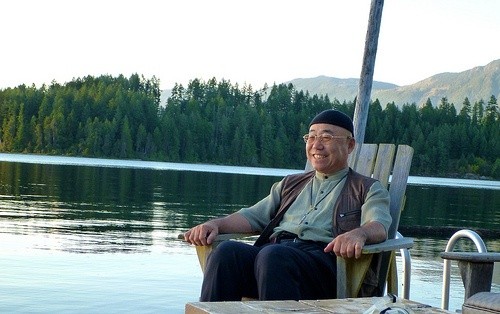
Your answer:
[178,143,414,299]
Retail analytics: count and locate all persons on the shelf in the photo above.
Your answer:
[184,109,392,300]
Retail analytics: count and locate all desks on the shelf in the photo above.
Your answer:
[185,296,448,314]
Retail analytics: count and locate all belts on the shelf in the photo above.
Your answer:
[271,238,314,244]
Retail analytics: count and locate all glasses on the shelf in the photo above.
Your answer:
[302,133,351,144]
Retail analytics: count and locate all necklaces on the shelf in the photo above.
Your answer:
[298,172,349,225]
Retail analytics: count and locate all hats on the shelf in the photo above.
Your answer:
[308,109,354,137]
